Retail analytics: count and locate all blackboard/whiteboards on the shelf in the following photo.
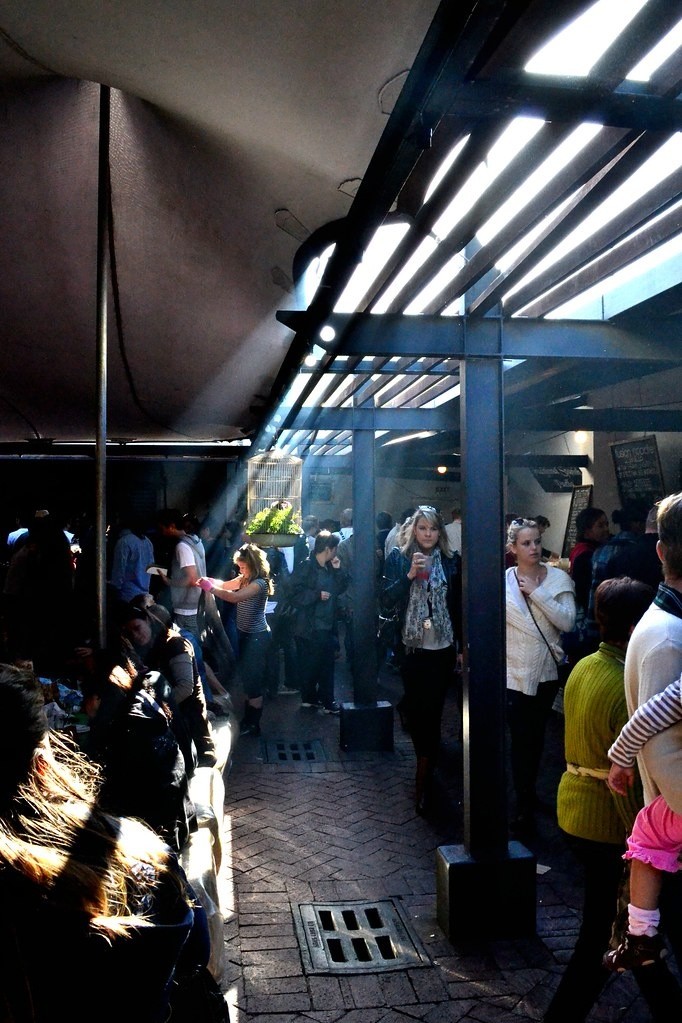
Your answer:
[561,484,593,558]
[529,466,583,493]
[306,480,335,506]
[608,434,666,511]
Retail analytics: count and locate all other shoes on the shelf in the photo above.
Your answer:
[324,701,340,714]
[278,684,300,695]
[603,933,670,971]
[301,695,321,707]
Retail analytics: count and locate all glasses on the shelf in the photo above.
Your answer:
[418,505,440,514]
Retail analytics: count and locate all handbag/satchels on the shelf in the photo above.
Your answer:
[379,547,406,632]
[557,655,574,689]
[266,601,294,644]
[170,694,207,741]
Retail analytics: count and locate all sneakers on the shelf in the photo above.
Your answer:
[512,774,554,835]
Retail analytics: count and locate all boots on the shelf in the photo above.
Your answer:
[414,756,436,813]
[239,700,264,737]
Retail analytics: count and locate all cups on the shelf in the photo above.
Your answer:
[415,555,433,580]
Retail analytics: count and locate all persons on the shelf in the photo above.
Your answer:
[0,506,273,1023]
[291,529,347,713]
[196,542,273,738]
[506,517,576,831]
[293,507,561,676]
[569,493,682,971]
[379,505,464,816]
[543,576,682,1023]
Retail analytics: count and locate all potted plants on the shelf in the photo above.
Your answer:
[245,506,306,547]
